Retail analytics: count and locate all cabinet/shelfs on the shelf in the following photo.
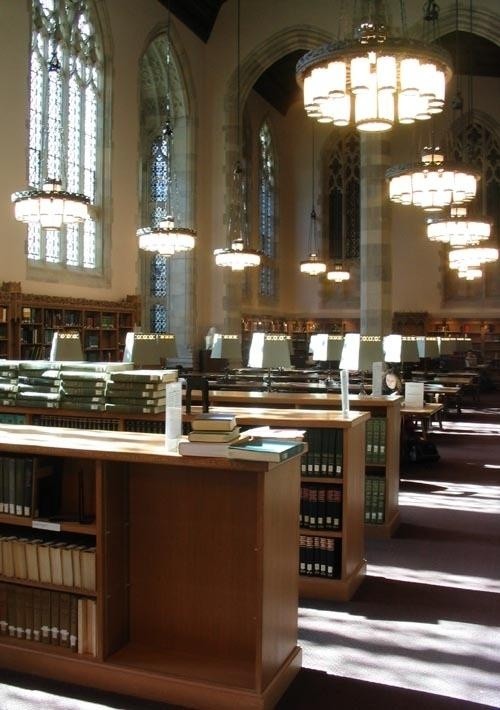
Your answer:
[1,281,147,368]
[234,307,499,370]
[2,420,320,708]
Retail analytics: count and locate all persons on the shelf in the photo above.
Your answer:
[382,369,403,394]
[465,350,477,366]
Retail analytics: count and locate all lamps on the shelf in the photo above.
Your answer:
[327,261,353,285]
[208,236,263,276]
[132,215,200,264]
[298,250,330,277]
[292,17,458,136]
[422,216,500,288]
[382,150,483,222]
[7,177,95,236]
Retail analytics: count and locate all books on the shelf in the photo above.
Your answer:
[1,356,179,433]
[298,427,340,578]
[366,416,385,522]
[176,412,303,463]
[5,452,98,657]
[1,305,124,362]
